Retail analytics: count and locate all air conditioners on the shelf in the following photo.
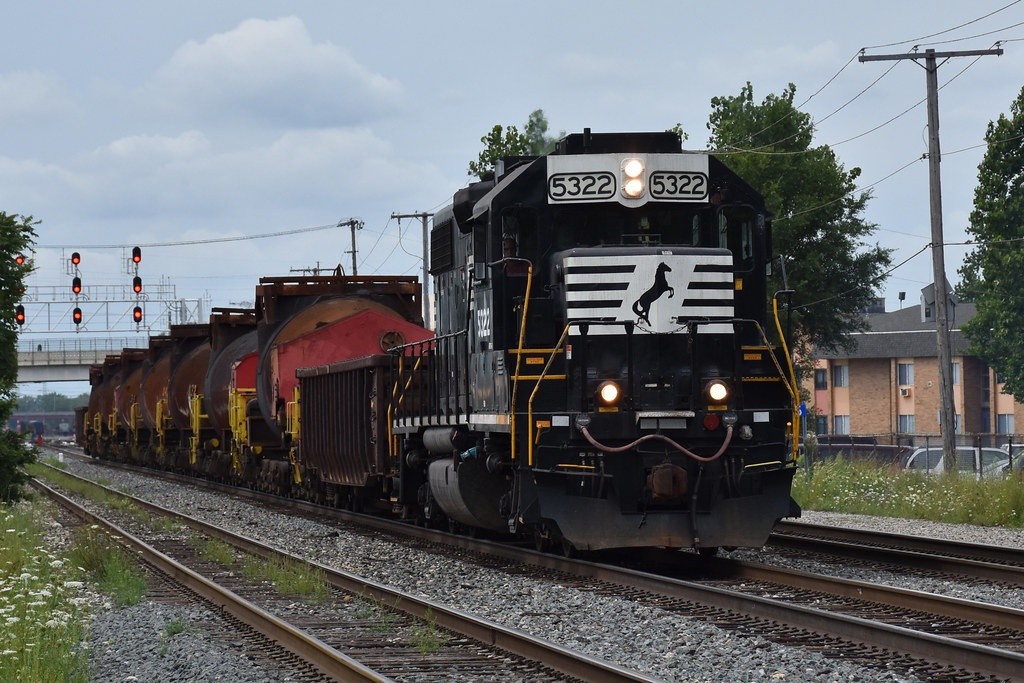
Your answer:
[900,388,910,396]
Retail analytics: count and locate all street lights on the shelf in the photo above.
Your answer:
[899,291,906,309]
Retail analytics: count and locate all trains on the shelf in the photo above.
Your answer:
[74,127,805,559]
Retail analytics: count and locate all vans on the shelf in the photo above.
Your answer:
[797,442,915,468]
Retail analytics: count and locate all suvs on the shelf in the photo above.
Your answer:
[900,442,1016,485]
[993,443,1024,480]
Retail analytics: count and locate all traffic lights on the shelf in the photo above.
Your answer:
[133,277,142,293]
[132,246,142,264]
[15,256,24,266]
[72,277,81,293]
[71,252,81,265]
[16,305,25,325]
[73,307,82,324]
[133,306,142,322]
